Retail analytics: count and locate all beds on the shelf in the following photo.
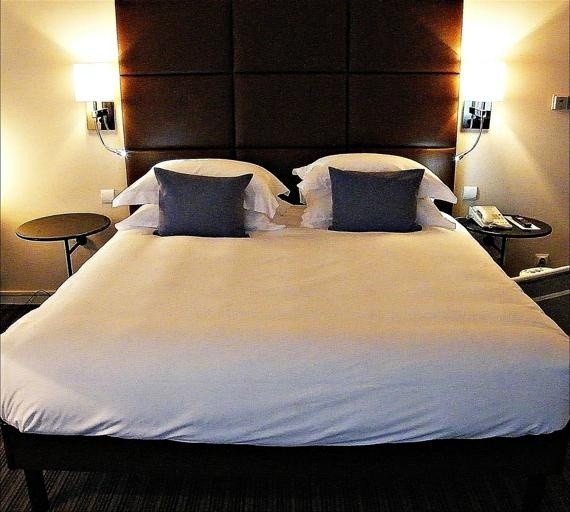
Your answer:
[1,203,570,510]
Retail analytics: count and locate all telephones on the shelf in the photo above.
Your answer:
[468,205,513,230]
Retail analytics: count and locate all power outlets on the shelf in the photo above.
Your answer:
[533,253,550,267]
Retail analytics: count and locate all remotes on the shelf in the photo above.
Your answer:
[512,216,531,227]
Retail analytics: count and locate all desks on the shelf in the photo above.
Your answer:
[14,212,111,277]
[458,213,552,275]
